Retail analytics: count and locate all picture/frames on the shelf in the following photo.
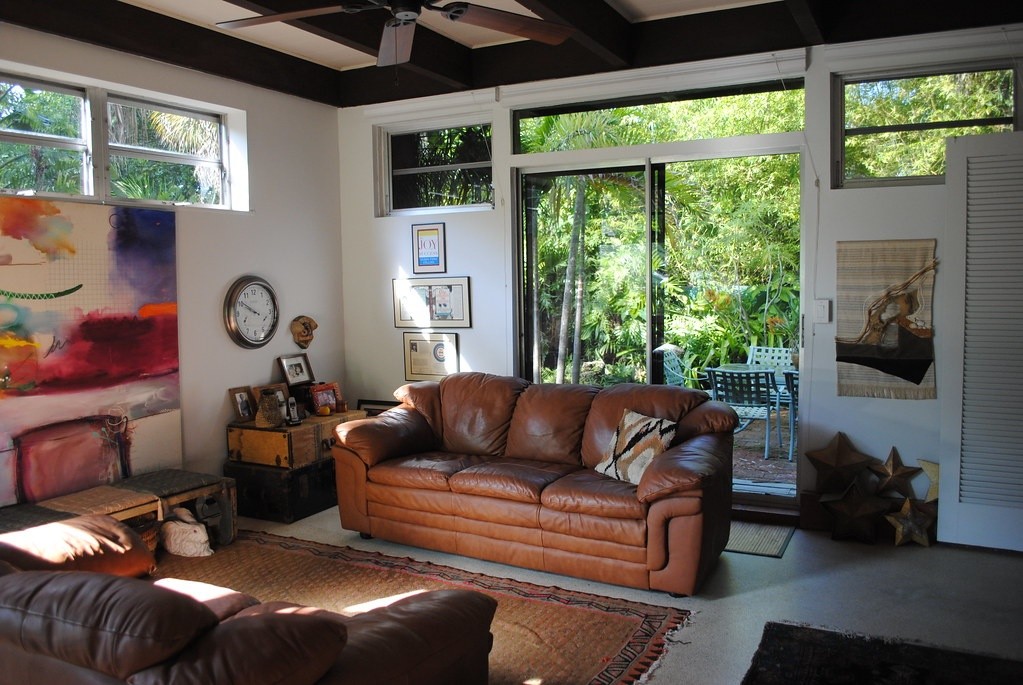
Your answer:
[392,276,472,329]
[252,382,289,406]
[310,383,342,416]
[402,331,461,382]
[229,386,259,423]
[277,352,315,387]
[411,222,446,275]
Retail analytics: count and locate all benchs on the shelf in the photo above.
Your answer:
[0,469,238,545]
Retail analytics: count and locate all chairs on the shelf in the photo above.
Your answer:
[660,346,799,462]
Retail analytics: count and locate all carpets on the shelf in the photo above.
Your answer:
[738,620,1023,685]
[722,516,799,559]
[146,528,691,684]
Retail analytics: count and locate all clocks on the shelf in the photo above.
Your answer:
[223,276,280,350]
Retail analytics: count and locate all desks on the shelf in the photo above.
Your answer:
[713,364,799,403]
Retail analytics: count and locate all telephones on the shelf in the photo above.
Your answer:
[285,397,301,427]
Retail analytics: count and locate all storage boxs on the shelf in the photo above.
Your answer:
[223,410,368,525]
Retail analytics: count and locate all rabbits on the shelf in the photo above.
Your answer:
[159,508,214,558]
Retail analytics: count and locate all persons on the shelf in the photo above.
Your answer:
[240,394,252,417]
[295,363,303,377]
[321,392,334,405]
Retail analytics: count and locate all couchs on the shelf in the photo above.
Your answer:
[0,512,498,685]
[331,372,740,598]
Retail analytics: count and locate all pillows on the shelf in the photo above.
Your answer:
[594,407,677,485]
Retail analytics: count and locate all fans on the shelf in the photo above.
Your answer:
[214,0,570,67]
